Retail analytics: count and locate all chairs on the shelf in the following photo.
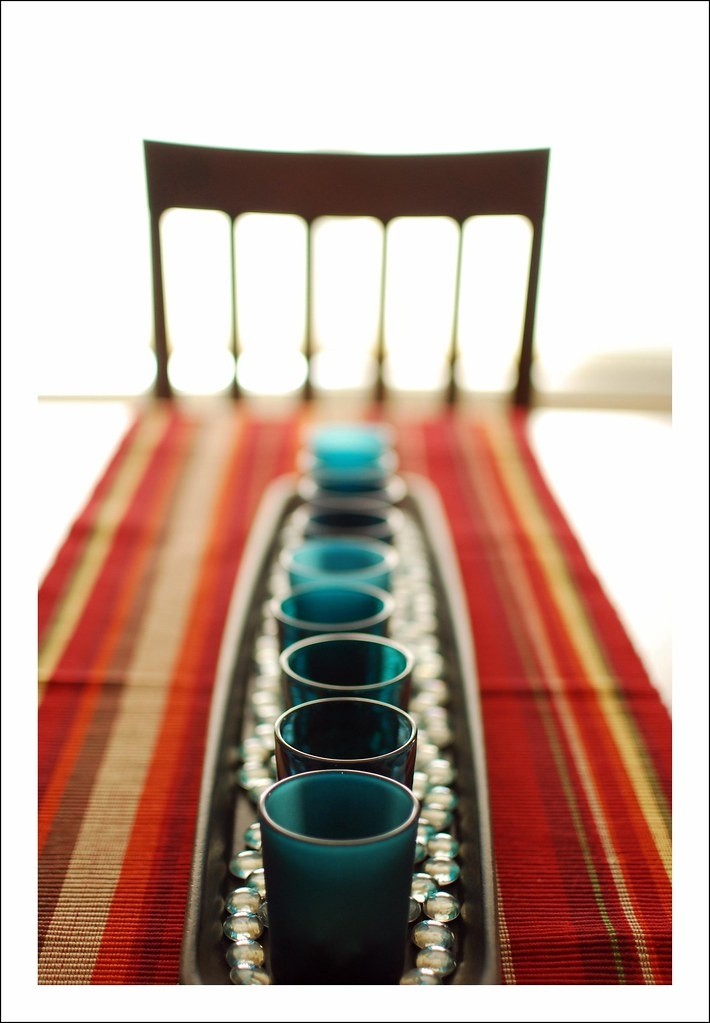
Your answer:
[141,138,552,407]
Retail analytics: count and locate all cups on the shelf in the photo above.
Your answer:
[279,632,415,713]
[279,420,398,594]
[274,696,419,792]
[271,581,396,652]
[258,769,420,986]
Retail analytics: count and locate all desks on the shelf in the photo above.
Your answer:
[38,391,672,985]
